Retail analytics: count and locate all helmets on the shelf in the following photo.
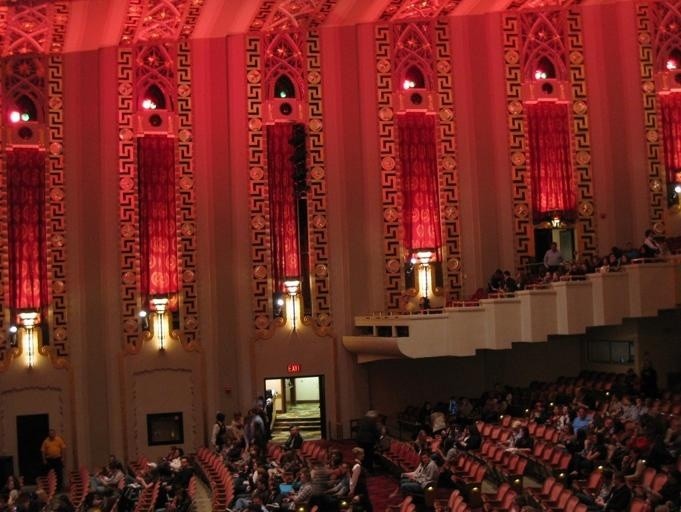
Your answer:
[217,412,224,419]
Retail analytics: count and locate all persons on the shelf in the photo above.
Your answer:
[2,355,678,511]
[419,297,432,314]
[472,228,681,307]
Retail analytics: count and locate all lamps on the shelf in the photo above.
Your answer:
[15,310,40,369]
[415,249,434,299]
[284,279,302,336]
[151,296,171,354]
